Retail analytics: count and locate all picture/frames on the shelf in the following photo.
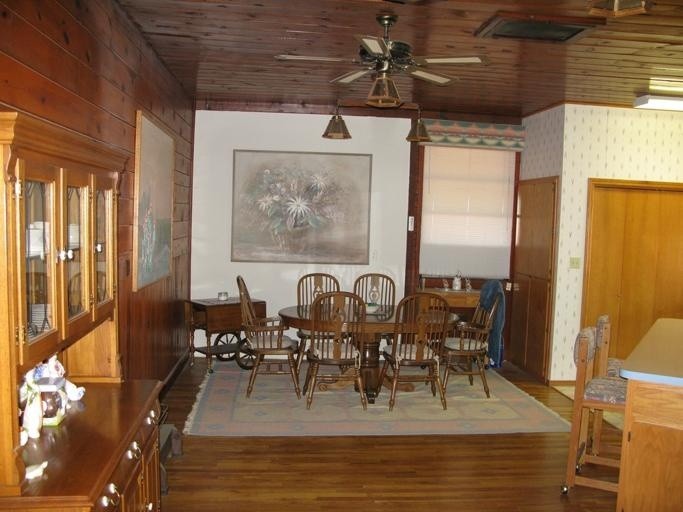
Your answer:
[230,149,375,265]
[130,107,177,293]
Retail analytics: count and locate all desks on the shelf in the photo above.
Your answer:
[616,317,682,512]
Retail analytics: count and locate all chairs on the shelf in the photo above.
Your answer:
[592,314,631,457]
[234,271,510,414]
[562,326,628,493]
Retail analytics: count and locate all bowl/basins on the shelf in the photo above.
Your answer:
[364,304,379,315]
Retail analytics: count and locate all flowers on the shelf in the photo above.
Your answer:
[237,163,346,232]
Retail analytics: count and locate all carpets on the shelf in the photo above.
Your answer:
[551,384,623,433]
[183,360,572,438]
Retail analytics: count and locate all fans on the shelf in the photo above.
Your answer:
[275,12,485,88]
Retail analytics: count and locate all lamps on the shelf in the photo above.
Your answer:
[406,103,434,143]
[322,101,350,139]
[633,94,682,110]
[366,71,401,107]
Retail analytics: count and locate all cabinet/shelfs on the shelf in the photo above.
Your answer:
[187,297,267,373]
[515,176,560,279]
[1,108,168,511]
[505,273,556,385]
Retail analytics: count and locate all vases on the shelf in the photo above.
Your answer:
[268,224,312,256]
